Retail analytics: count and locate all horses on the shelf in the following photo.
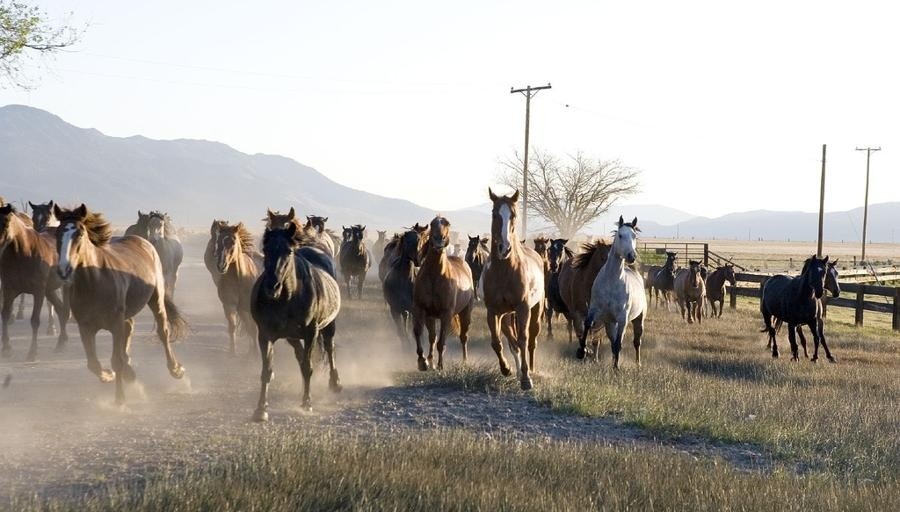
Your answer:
[375,213,489,377]
[648,251,677,312]
[773,258,840,363]
[486,187,545,391]
[673,266,710,318]
[520,215,647,377]
[0,196,194,411]
[706,263,735,319]
[206,207,374,421]
[760,254,829,363]
[674,259,705,325]
